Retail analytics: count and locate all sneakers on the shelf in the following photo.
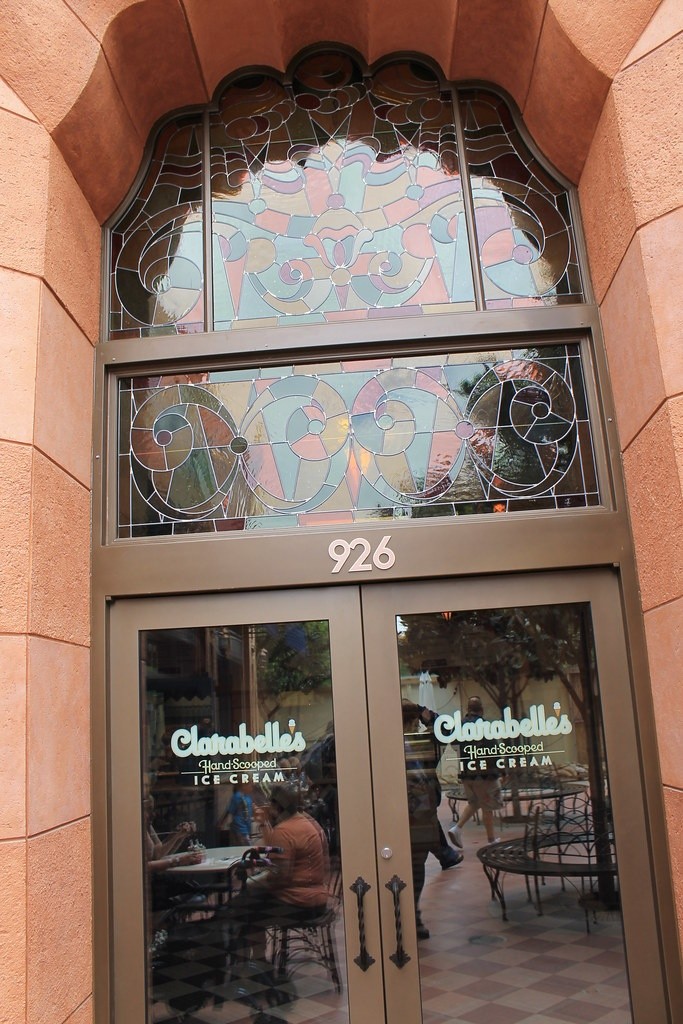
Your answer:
[489,837,501,845]
[448,825,464,848]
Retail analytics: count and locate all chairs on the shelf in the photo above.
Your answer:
[271,864,348,991]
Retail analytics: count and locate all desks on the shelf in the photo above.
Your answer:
[157,846,268,983]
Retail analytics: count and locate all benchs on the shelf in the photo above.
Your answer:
[446,764,588,823]
[477,791,619,935]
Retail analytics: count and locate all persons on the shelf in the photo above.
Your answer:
[429,819,464,870]
[306,721,341,855]
[402,698,456,939]
[141,769,331,975]
[447,697,506,848]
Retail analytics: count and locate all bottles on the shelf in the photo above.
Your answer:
[289,772,307,792]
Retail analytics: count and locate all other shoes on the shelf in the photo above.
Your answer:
[414,919,430,939]
[442,855,464,869]
